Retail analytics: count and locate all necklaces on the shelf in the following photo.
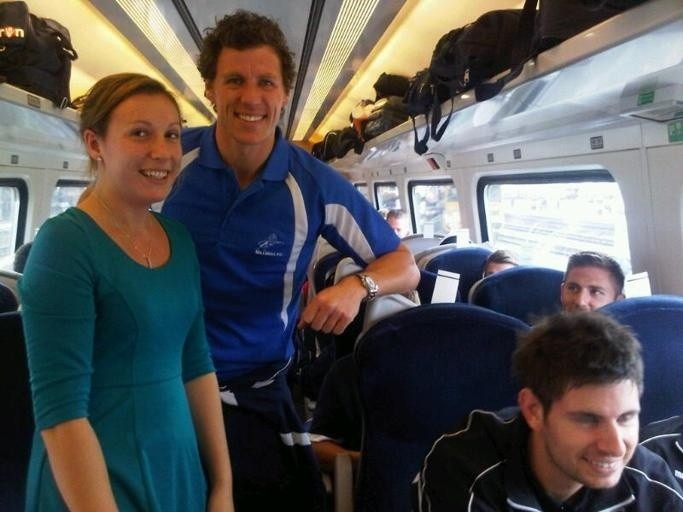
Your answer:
[92,192,153,269]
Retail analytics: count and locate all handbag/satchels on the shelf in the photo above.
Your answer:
[1,1,79,111]
[307,0,648,160]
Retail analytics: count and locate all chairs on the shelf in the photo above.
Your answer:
[0,242,33,512]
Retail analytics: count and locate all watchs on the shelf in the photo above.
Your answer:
[355,272,379,301]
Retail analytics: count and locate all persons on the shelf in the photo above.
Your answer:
[77,7,421,512]
[559,251,625,312]
[15,71,238,511]
[402,309,683,512]
[385,208,411,239]
[480,248,519,279]
